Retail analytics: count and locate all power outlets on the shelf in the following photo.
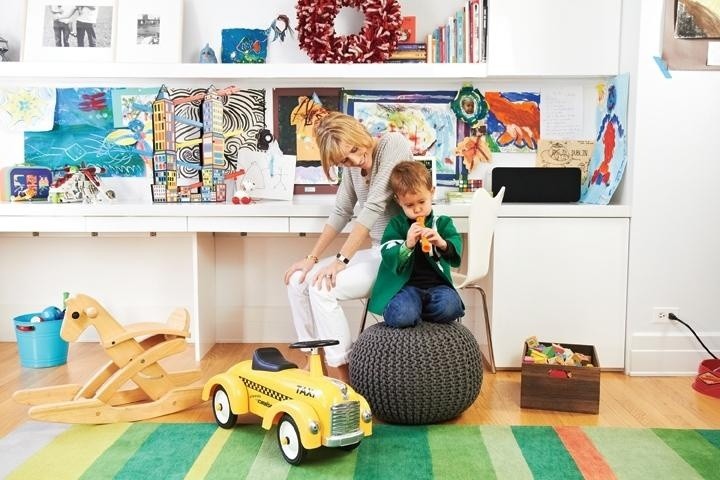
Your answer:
[650,307,679,325]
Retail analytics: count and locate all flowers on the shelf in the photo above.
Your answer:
[295,0,403,64]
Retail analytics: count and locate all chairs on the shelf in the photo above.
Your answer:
[352,184,510,372]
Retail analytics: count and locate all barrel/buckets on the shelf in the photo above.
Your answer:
[13,312,69,369]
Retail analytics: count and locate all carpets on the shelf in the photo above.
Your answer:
[0,417,718,480]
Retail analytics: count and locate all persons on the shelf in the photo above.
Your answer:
[463,99,475,115]
[365,161,465,330]
[50,4,101,47]
[284,111,414,384]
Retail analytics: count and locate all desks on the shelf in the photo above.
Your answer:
[1,201,632,375]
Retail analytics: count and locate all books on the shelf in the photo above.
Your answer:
[383,0,488,64]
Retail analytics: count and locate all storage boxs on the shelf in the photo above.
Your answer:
[520,341,601,415]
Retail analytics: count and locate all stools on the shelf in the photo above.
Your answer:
[346,320,483,427]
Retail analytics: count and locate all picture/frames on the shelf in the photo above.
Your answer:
[1,1,186,66]
[273,87,345,195]
